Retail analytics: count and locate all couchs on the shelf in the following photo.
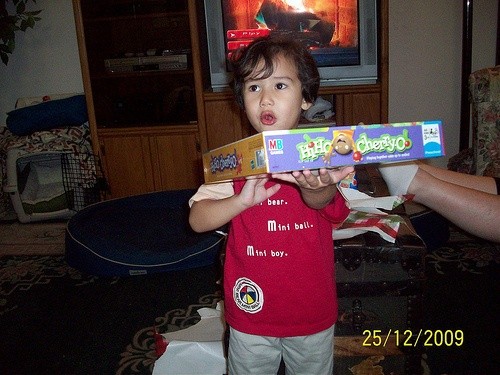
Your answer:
[403,66,500,375]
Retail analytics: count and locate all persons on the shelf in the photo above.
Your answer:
[379,160,500,243]
[187,35,355,375]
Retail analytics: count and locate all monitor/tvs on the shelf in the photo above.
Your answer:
[204,0,377,93]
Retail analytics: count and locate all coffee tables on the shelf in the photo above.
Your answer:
[219,163,427,375]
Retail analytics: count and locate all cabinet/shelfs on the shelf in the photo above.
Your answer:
[71,0,388,203]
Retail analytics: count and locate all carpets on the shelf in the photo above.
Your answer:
[0,244,227,375]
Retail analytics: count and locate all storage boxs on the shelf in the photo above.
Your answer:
[201,119,444,186]
